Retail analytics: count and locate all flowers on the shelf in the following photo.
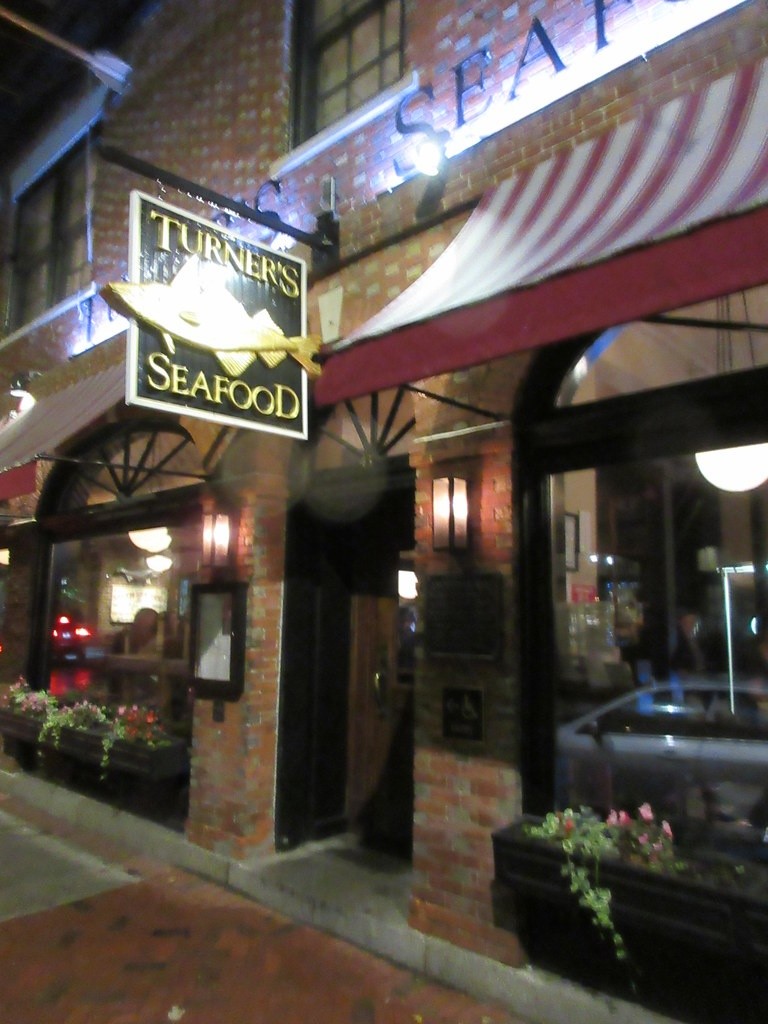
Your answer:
[523,803,672,960]
[0,673,158,780]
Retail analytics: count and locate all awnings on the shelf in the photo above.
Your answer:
[316,59,768,406]
[0,357,131,500]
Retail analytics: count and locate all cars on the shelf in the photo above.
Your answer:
[52,617,90,663]
[553,682,768,822]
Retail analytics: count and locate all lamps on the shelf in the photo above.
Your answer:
[202,512,232,567]
[695,290,768,492]
[432,466,474,552]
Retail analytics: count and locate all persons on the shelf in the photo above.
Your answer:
[111,607,193,719]
[394,606,416,704]
[670,613,716,678]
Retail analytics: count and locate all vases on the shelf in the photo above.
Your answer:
[492,817,768,969]
[0,708,187,782]
